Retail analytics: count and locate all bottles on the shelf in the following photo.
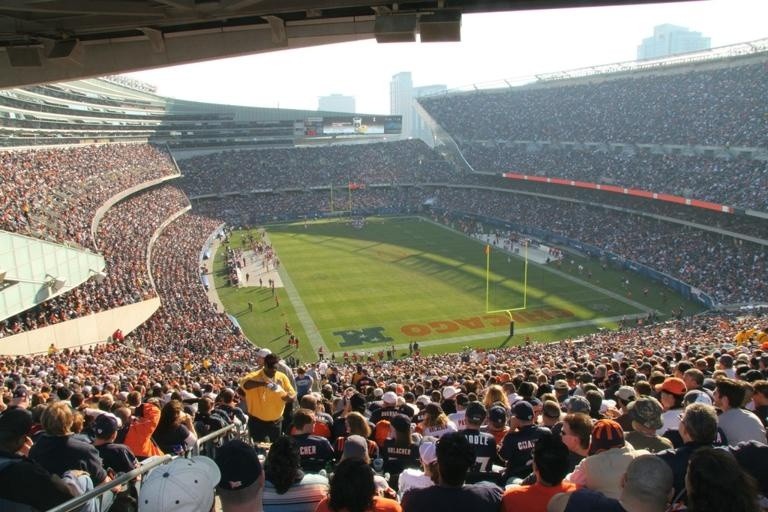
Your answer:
[266,382,285,395]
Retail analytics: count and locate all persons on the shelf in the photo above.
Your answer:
[283,129,448,230]
[417,49,766,338]
[262,324,766,510]
[0,82,296,512]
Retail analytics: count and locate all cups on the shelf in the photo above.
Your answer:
[373,459,384,472]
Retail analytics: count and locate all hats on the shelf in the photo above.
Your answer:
[554,380,568,389]
[139,441,260,512]
[255,349,271,358]
[591,378,688,448]
[96,414,122,430]
[466,400,561,424]
[374,385,461,404]
